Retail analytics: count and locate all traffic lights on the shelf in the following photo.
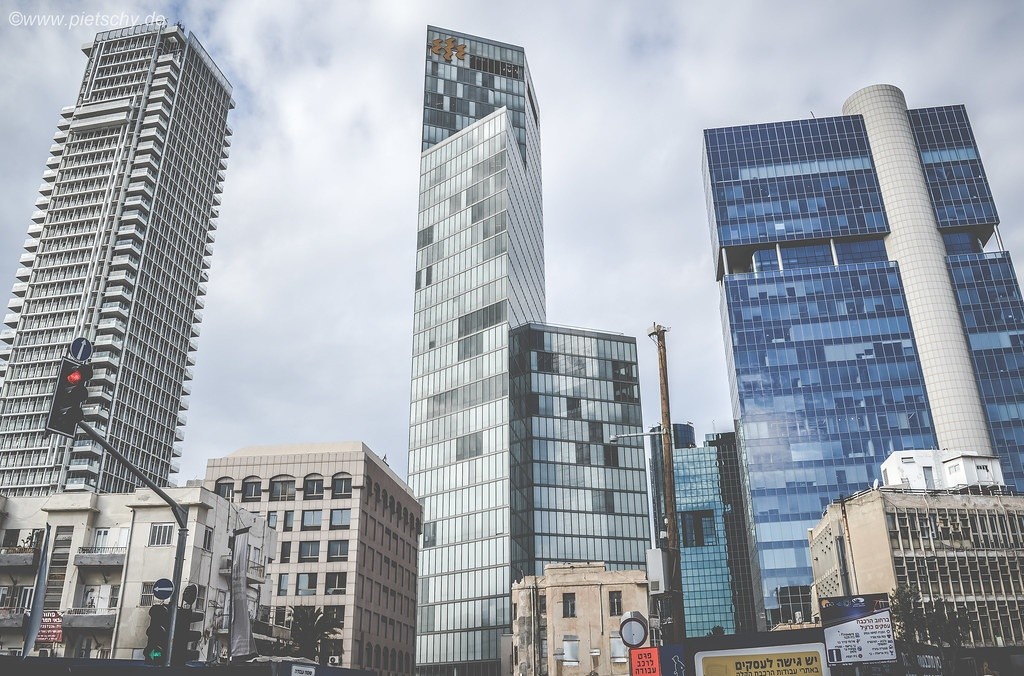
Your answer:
[44,357,93,438]
[172,609,204,662]
[142,603,170,664]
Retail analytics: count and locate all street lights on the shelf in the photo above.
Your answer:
[610,323,688,639]
